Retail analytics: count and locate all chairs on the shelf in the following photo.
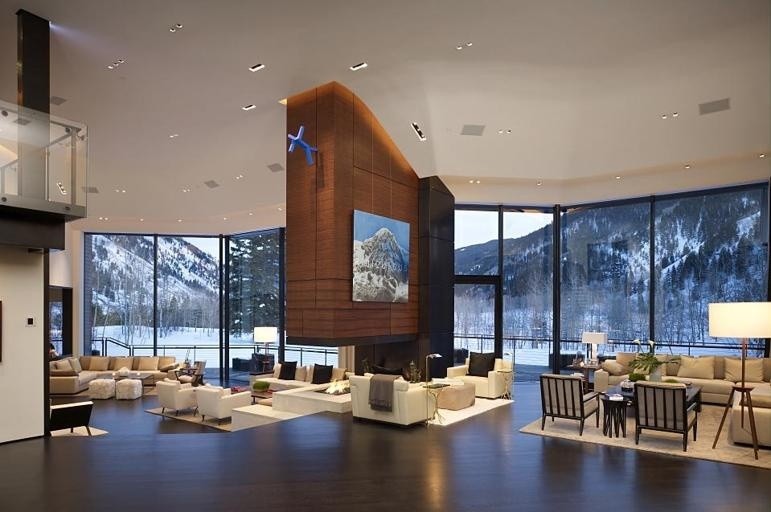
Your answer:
[537,373,599,438]
[634,381,699,453]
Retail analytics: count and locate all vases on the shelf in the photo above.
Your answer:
[649,367,663,382]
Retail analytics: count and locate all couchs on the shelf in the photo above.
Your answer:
[355,334,437,383]
[592,351,770,449]
[250,365,346,393]
[351,372,435,427]
[446,357,510,401]
[50,349,254,436]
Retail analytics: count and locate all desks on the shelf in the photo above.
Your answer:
[421,378,474,410]
[601,394,629,440]
[565,364,601,394]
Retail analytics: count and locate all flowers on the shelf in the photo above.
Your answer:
[628,339,681,373]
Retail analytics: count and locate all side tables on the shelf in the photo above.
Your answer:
[421,383,450,428]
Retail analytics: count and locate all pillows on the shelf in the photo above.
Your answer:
[633,352,668,376]
[279,360,297,380]
[722,355,763,383]
[601,359,633,377]
[469,351,497,377]
[674,354,715,383]
[312,363,332,386]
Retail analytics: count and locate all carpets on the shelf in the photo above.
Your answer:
[426,397,512,427]
[518,391,770,471]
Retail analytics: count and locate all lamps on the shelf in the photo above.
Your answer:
[253,327,278,374]
[708,302,771,459]
[582,332,608,365]
